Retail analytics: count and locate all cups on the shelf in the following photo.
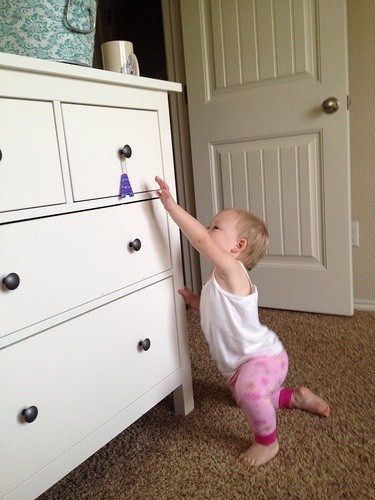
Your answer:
[101,41,139,76]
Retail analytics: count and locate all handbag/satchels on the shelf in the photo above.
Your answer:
[1,0,97,68]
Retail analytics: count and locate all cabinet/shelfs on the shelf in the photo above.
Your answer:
[0,52,194,500]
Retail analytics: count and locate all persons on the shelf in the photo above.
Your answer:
[155,175,331,468]
